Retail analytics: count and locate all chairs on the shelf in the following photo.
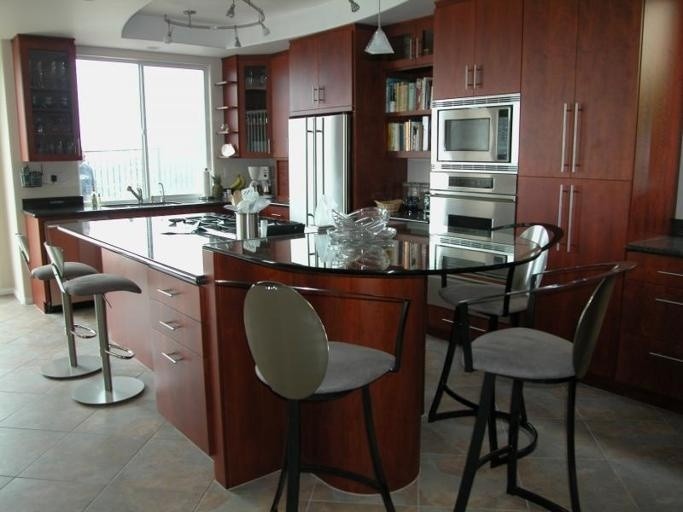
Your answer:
[452,261,638,512]
[214,279,411,512]
[428,222,564,468]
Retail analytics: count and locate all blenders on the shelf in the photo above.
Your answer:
[401,181,422,220]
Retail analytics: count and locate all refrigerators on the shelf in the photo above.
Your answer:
[288,227,349,270]
[288,113,350,227]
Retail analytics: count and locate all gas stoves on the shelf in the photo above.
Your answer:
[168,214,305,240]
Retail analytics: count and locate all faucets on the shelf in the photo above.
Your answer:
[126,185,143,203]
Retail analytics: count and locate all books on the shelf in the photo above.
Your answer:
[384,75,434,152]
[409,28,434,61]
[390,239,429,270]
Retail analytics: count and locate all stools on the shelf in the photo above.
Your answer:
[43,240,144,408]
[15,232,103,380]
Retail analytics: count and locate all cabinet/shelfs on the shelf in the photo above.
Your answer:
[10,34,82,163]
[271,49,289,202]
[289,22,389,211]
[23,216,101,314]
[432,1,523,98]
[99,248,212,460]
[515,1,683,392]
[617,252,682,415]
[214,54,271,159]
[384,13,433,161]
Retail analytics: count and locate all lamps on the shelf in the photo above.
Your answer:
[165,21,172,43]
[348,0,361,13]
[365,1,395,54]
[184,10,197,29]
[258,19,271,36]
[227,0,236,16]
[233,26,242,47]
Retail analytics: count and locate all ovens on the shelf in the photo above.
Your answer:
[429,224,515,271]
[427,93,521,324]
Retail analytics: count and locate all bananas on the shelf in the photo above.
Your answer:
[229,175,246,189]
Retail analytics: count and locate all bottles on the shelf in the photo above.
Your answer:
[96,193,101,210]
[227,189,231,197]
[222,192,227,198]
[27,58,76,155]
[92,193,97,210]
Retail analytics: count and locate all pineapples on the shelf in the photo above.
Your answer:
[211,175,223,198]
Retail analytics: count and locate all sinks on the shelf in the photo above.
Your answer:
[99,201,185,209]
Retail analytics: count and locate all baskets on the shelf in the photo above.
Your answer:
[374,200,402,213]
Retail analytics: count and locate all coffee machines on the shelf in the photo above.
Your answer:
[248,166,271,196]
